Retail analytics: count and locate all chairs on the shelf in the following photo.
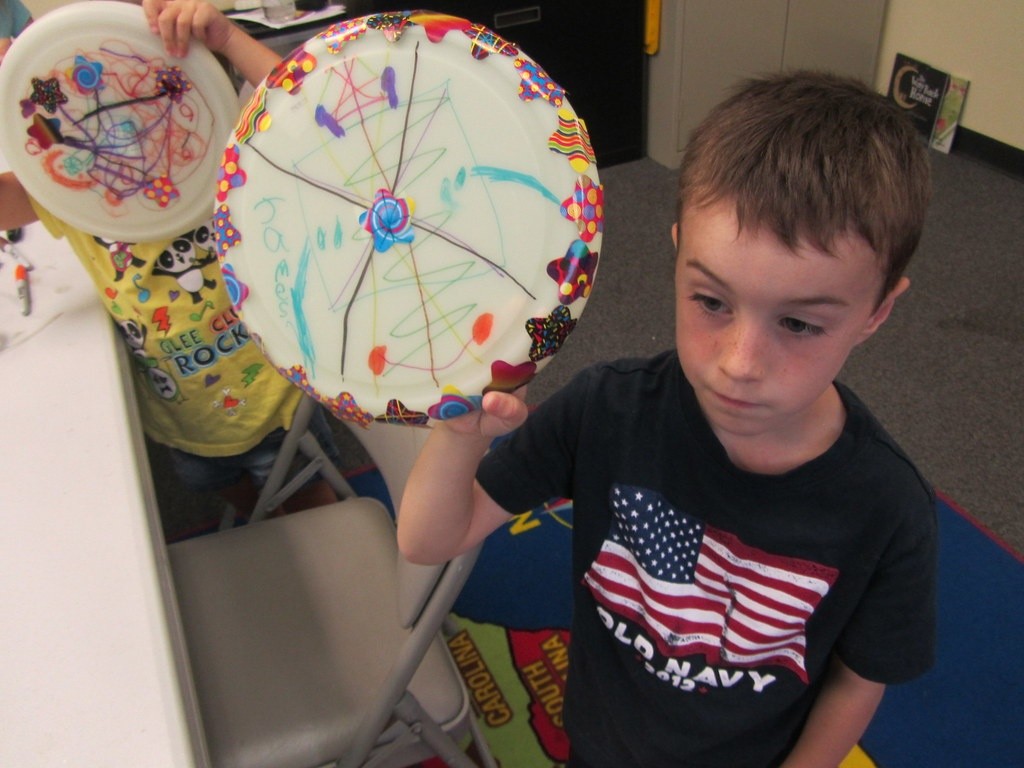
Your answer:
[184,392,481,768]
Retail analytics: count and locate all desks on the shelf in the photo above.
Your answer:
[0,234,212,768]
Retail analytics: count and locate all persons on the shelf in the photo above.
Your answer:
[0,0,338,542]
[395,69,938,768]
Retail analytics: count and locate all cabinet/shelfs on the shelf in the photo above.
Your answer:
[644,0,886,171]
[352,0,648,170]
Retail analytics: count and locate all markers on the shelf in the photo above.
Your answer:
[14,265,30,317]
[6,227,23,242]
[0,236,32,270]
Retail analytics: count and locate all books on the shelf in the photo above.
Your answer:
[886,51,971,154]
